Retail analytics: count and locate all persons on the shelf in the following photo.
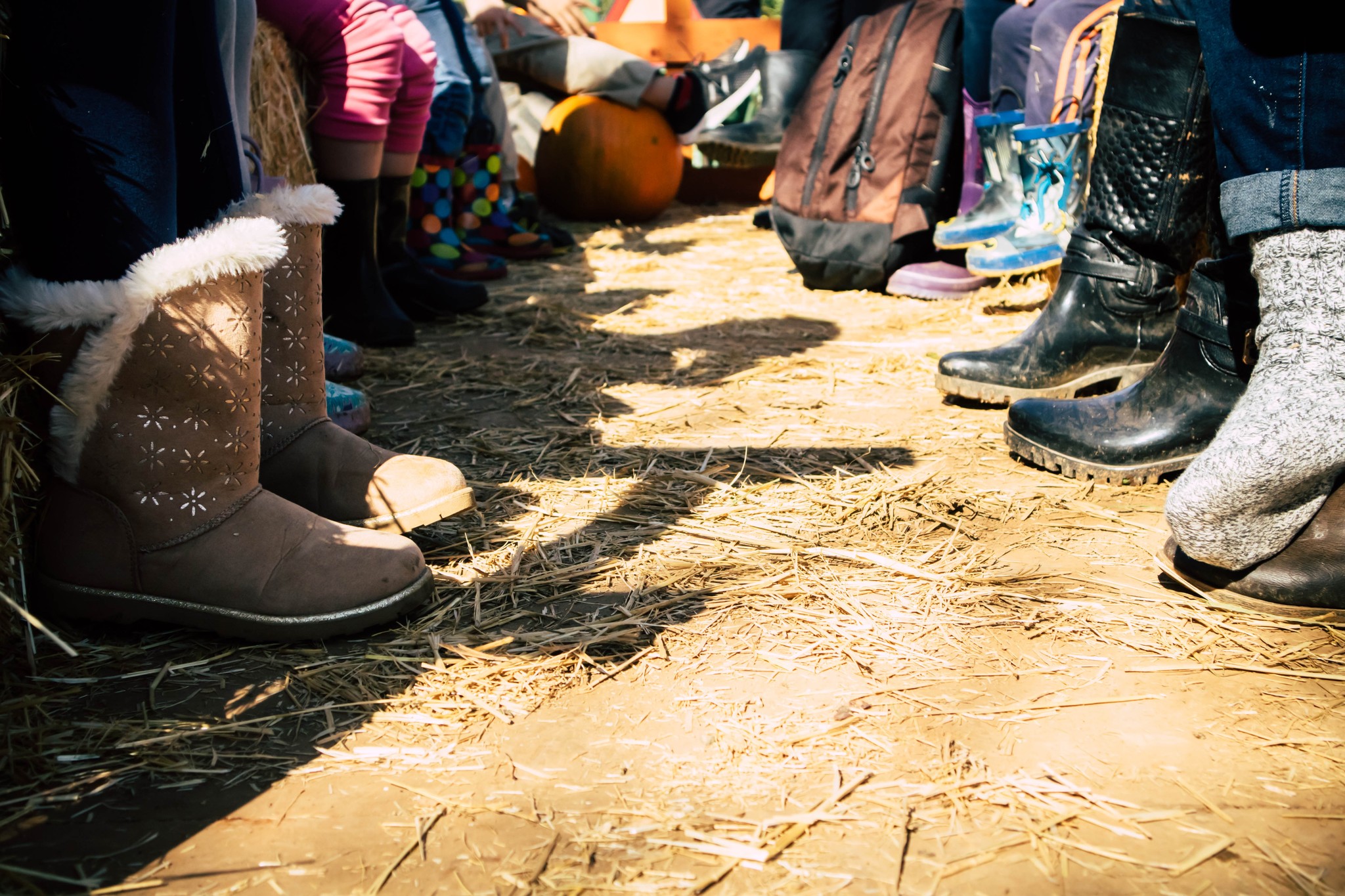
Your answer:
[0,0,1262,483]
[0,0,479,645]
[1155,1,1344,623]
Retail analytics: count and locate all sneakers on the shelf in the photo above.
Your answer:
[669,44,770,145]
[687,38,749,76]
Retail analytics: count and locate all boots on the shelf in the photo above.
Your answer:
[932,86,1026,249]
[935,232,1182,405]
[452,143,551,261]
[321,176,418,345]
[2,213,436,638]
[506,181,575,253]
[886,88,993,301]
[215,183,476,535]
[1003,258,1258,487]
[965,96,1094,277]
[412,155,507,280]
[377,174,489,316]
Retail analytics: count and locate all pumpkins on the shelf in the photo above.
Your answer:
[532,93,682,221]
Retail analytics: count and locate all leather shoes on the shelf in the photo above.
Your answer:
[1155,468,1345,624]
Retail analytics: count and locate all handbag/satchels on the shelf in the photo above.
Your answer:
[770,0,966,292]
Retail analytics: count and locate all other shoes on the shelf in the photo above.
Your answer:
[321,375,371,435]
[319,327,365,379]
